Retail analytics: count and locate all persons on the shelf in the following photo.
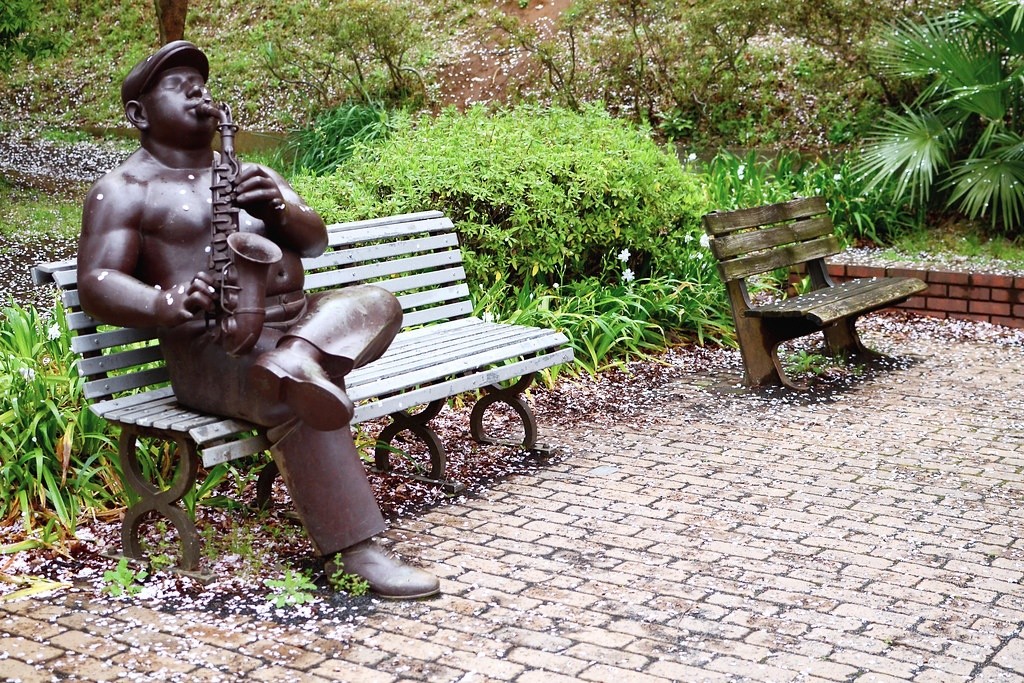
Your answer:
[77,41,441,601]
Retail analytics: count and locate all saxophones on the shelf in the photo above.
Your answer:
[195,94,283,360]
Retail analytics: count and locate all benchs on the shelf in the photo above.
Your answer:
[701,195,928,393]
[29,209,575,569]
